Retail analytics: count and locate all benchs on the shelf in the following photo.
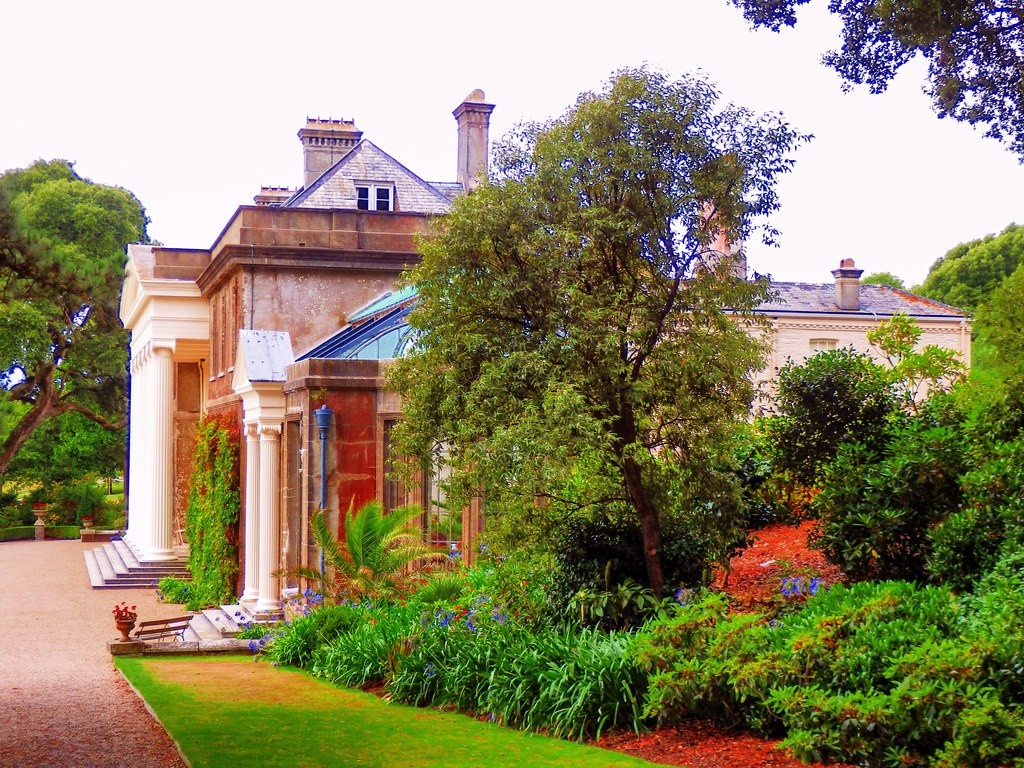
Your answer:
[114,616,193,643]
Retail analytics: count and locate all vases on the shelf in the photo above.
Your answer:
[31,510,48,525]
[83,522,93,530]
[115,621,135,642]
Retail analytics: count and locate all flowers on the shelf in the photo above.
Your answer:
[31,502,46,510]
[80,514,94,523]
[112,601,137,620]
